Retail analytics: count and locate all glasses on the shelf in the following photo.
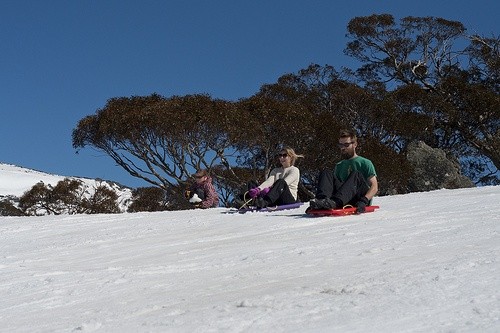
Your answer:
[195,174,205,178]
[278,153,289,158]
[339,140,353,147]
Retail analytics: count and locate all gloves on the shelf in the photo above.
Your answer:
[355,195,369,212]
[249,187,260,197]
[261,187,272,195]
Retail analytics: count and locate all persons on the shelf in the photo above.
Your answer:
[305,131,379,214]
[245,147,304,211]
[180,169,220,209]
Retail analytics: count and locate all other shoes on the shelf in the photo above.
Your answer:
[256,196,265,209]
[235,198,250,209]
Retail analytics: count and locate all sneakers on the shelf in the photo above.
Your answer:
[321,197,337,209]
[310,197,326,209]
